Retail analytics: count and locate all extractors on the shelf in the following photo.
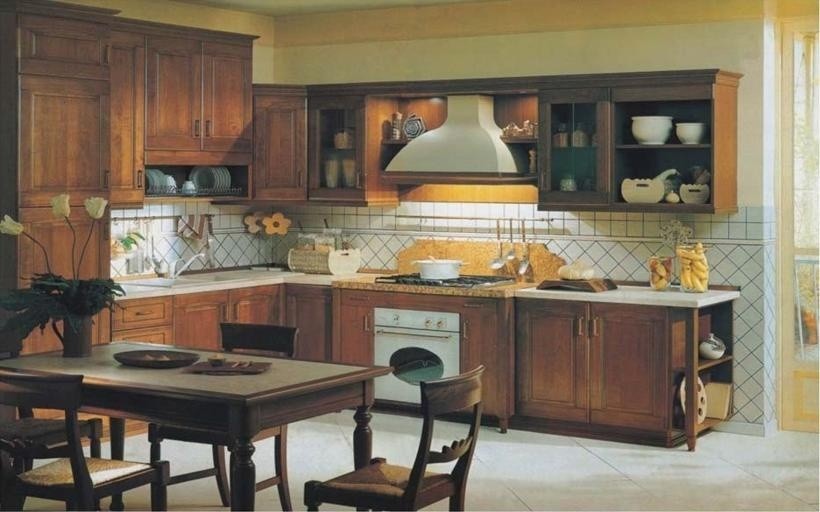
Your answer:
[383,94,535,187]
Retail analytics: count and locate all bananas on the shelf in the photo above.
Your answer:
[675,241,709,292]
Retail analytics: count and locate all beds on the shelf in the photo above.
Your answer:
[0,338,395,512]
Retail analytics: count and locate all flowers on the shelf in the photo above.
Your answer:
[0,193,126,342]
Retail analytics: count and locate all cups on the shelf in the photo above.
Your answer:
[343,160,357,187]
[324,160,338,188]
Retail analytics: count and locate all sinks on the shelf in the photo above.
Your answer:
[136,272,252,288]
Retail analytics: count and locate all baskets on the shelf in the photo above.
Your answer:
[286,247,361,275]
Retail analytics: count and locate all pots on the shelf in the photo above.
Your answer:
[412,257,468,280]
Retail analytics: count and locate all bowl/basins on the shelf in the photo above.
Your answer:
[182,180,197,194]
[676,123,706,145]
[631,115,672,146]
[208,357,226,366]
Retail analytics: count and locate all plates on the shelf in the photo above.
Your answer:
[189,167,232,194]
[114,349,200,369]
[145,169,166,195]
[188,361,272,375]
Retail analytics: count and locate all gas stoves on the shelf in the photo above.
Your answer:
[375,273,516,288]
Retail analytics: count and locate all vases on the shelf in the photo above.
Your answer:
[60,316,94,359]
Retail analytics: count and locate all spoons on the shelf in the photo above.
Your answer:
[490,220,504,270]
[507,220,515,261]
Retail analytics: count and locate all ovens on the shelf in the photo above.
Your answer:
[373,307,460,407]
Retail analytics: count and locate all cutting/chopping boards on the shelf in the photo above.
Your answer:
[537,278,617,292]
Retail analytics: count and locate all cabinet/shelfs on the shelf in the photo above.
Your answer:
[148,20,262,203]
[363,75,540,211]
[333,289,515,434]
[173,284,286,355]
[252,82,308,202]
[513,297,734,449]
[307,82,363,206]
[111,296,172,345]
[15,205,111,357]
[611,68,745,215]
[17,0,122,205]
[538,72,612,211]
[111,15,144,210]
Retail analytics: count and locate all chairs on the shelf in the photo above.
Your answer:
[0,370,171,512]
[302,366,486,512]
[0,327,103,512]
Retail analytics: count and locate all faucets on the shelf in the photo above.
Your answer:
[168,252,205,278]
